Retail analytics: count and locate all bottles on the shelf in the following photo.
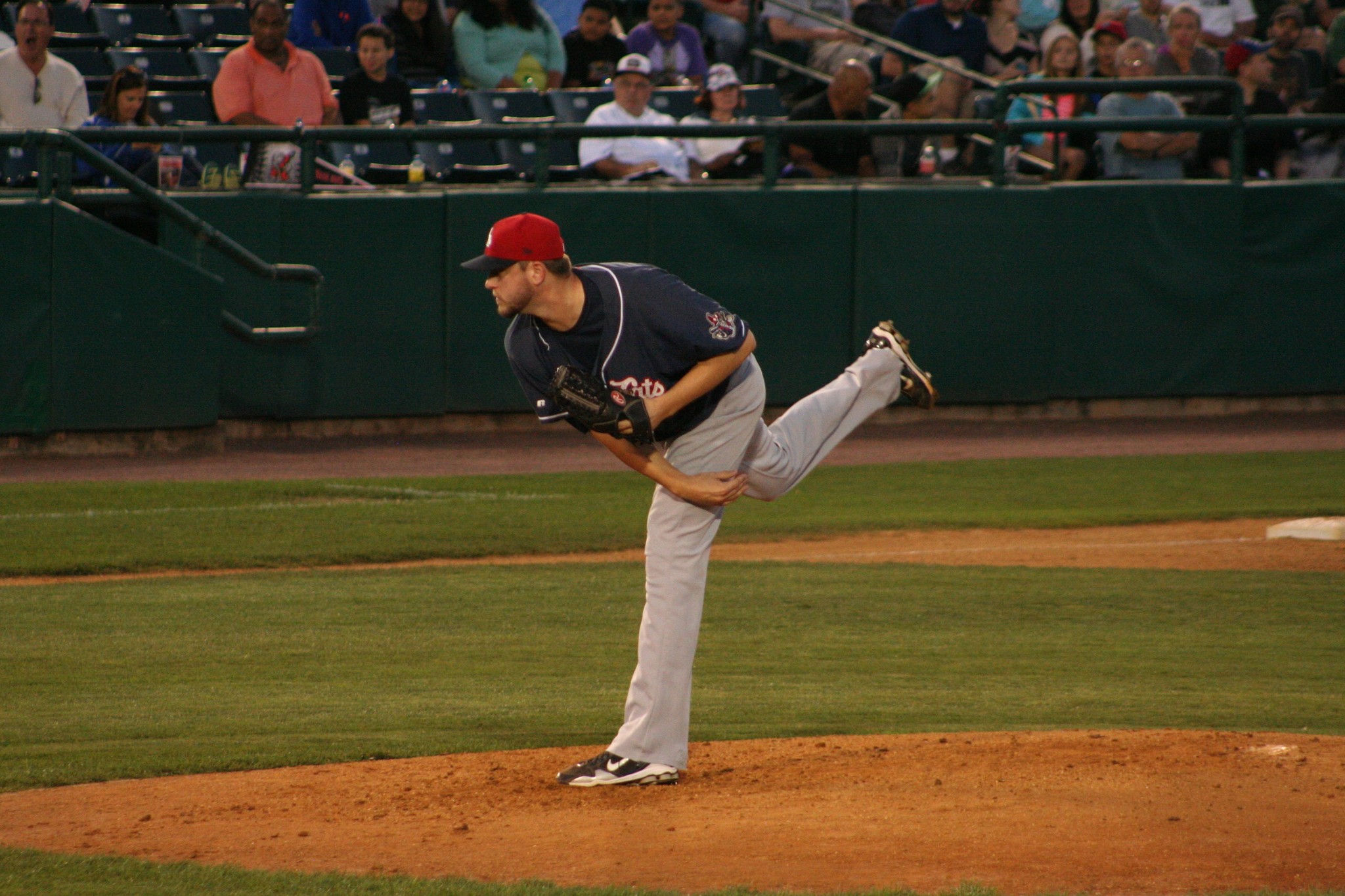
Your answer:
[600,76,612,88]
[339,154,355,175]
[438,77,451,90]
[920,146,936,186]
[521,75,535,89]
[408,154,425,184]
[677,74,693,86]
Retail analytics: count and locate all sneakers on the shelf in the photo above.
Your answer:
[556,749,678,786]
[866,321,936,410]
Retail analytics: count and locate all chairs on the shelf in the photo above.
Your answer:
[0,0,788,176]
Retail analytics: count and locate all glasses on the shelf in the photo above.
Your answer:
[33,77,42,105]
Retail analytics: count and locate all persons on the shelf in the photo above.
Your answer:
[0,0,1345,184]
[462,211,934,788]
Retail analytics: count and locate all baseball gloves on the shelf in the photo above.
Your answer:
[545,363,651,445]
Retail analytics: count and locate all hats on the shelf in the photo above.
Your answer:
[613,53,655,82]
[1038,23,1080,56]
[460,214,566,272]
[1224,34,1276,71]
[704,63,740,91]
[1090,20,1128,43]
